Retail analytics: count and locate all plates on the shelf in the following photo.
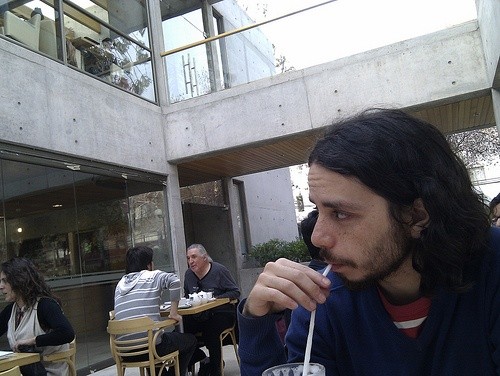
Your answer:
[160,305,171,310]
[209,298,216,302]
[0,351,14,357]
[178,304,190,309]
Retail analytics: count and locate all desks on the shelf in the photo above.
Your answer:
[73,35,116,79]
[0,352,40,371]
[160,298,231,376]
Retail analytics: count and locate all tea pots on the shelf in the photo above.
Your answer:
[187,292,203,307]
[199,291,210,304]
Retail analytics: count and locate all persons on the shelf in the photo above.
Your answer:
[237,103,500,376]
[182,243,241,376]
[114,246,197,376]
[0,256,74,376]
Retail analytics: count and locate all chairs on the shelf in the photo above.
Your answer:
[40,19,82,69]
[0,11,42,51]
[196,298,240,376]
[106,316,180,376]
[43,336,78,376]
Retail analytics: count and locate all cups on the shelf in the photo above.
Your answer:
[179,298,187,305]
[262,362,326,376]
[206,292,212,298]
